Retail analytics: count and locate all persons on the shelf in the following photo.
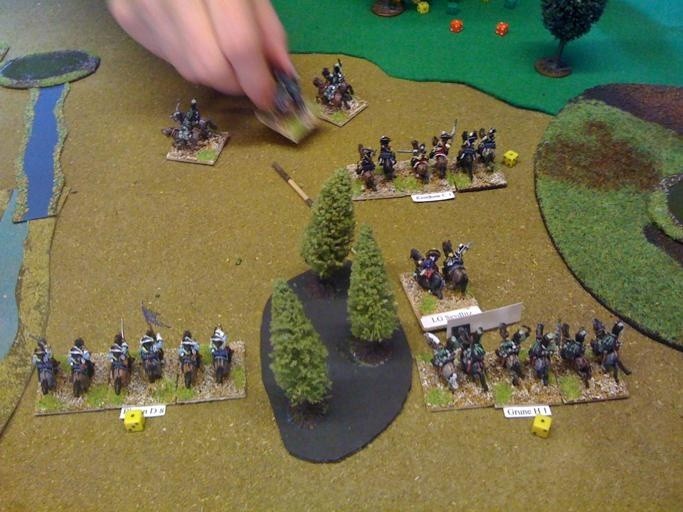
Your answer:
[408,247,445,300]
[416,317,632,396]
[440,240,471,296]
[311,58,368,128]
[161,97,229,167]
[100,0,302,112]
[352,117,498,194]
[21,303,247,399]
